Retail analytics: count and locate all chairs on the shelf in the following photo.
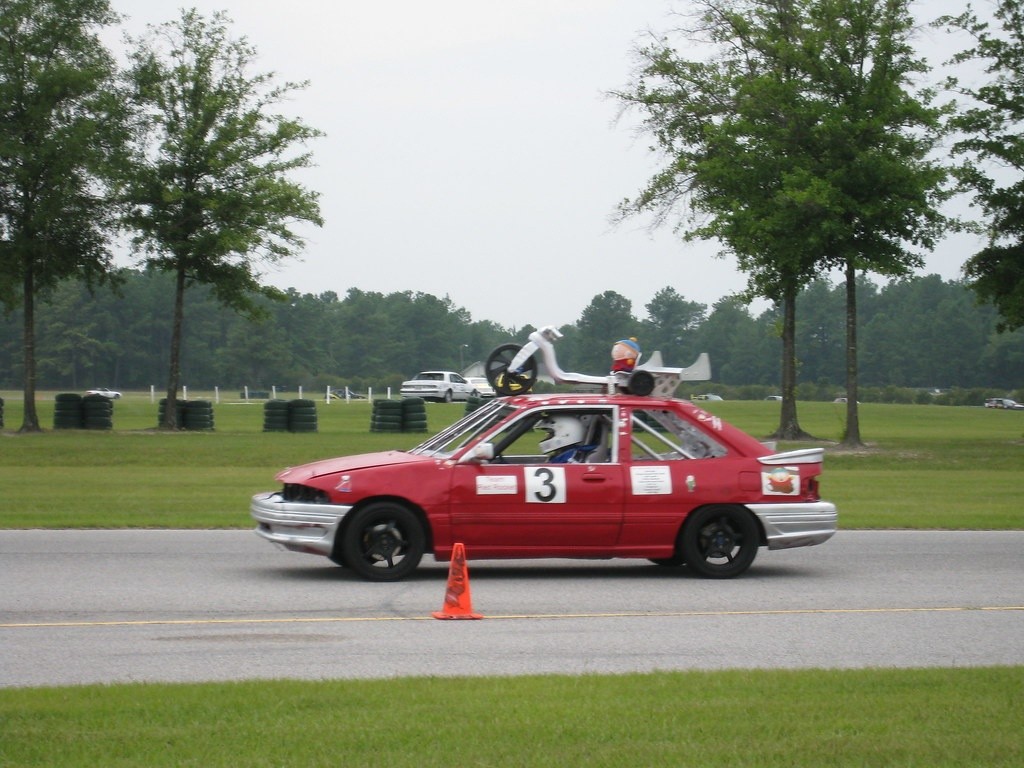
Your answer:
[583,445,608,463]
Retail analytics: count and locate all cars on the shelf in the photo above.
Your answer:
[326,388,366,399]
[400,371,478,403]
[86,386,122,399]
[696,395,723,400]
[251,393,838,579]
[834,398,861,404]
[764,395,783,400]
[463,375,498,399]
[985,398,1024,410]
[494,371,535,394]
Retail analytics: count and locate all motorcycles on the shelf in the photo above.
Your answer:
[483,327,710,395]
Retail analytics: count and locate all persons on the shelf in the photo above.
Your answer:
[612,337,639,372]
[533,414,586,463]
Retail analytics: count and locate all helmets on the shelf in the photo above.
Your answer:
[532,414,587,453]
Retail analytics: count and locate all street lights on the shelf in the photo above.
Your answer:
[461,343,469,376]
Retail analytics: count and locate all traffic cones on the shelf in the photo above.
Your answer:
[432,543,485,620]
